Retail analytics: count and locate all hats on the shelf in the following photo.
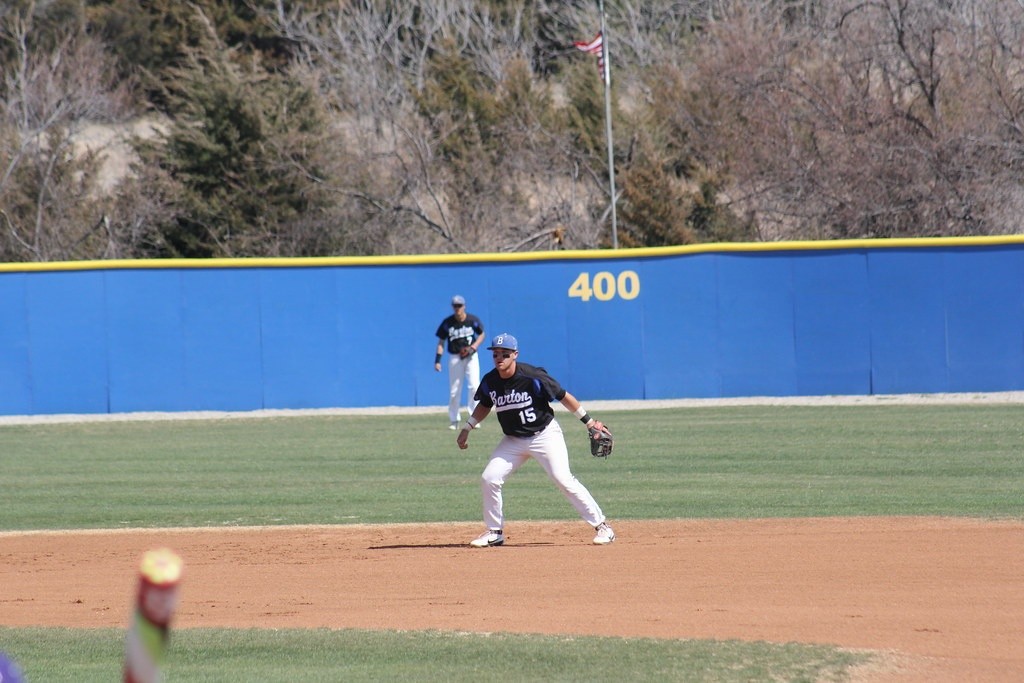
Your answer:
[451,295,465,305]
[487,332,518,351]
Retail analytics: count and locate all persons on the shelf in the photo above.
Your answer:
[456,334,615,548]
[435,295,485,430]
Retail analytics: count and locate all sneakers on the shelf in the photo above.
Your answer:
[470,530,504,548]
[593,524,615,545]
[473,423,480,429]
[449,422,459,430]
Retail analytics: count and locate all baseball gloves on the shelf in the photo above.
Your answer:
[588,420,613,457]
[460,345,476,359]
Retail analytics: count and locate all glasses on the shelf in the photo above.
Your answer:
[492,352,514,358]
[453,305,463,308]
